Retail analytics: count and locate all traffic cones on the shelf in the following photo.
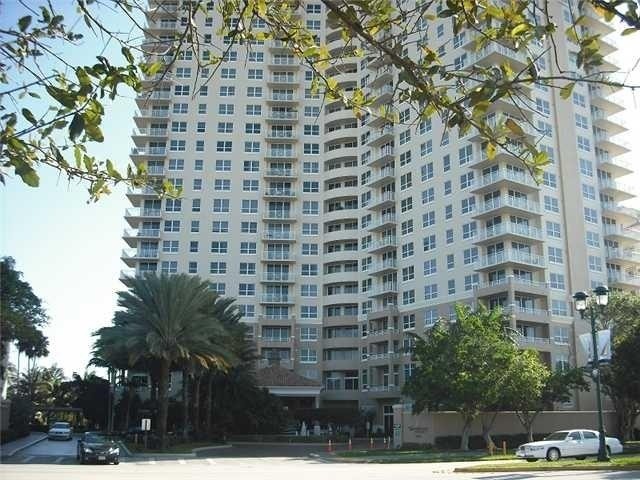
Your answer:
[348,439,352,450]
[383,437,387,447]
[328,439,332,451]
[370,438,374,448]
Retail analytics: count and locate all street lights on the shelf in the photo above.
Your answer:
[516,428,623,462]
[572,285,611,462]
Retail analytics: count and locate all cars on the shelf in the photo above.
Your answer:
[48,422,74,440]
[76,430,120,465]
